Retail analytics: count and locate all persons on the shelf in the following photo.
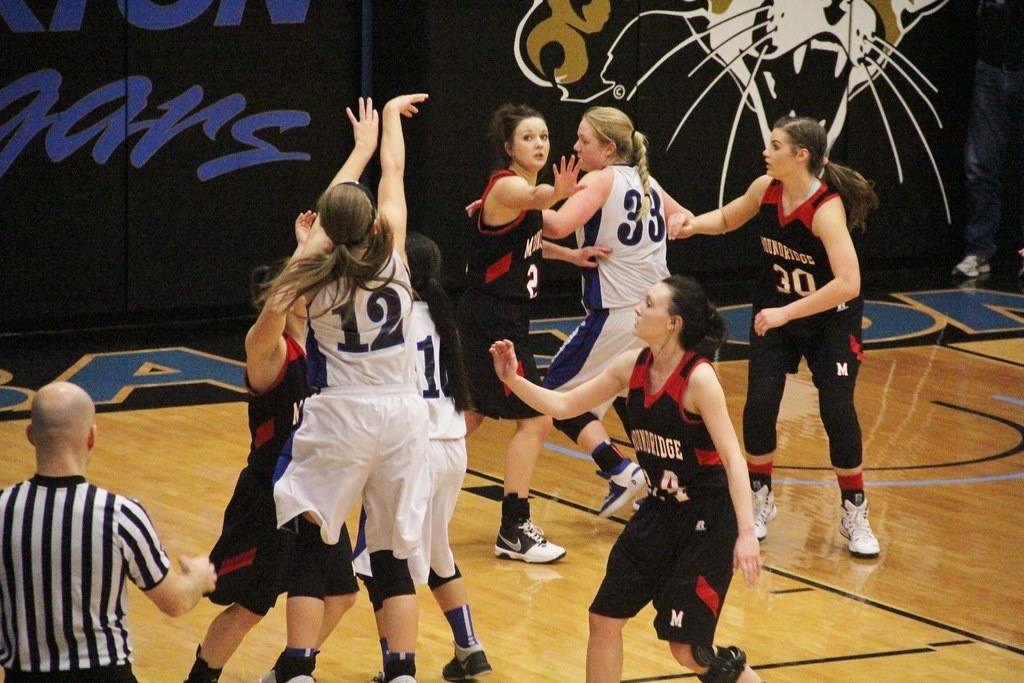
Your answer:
[489,275,769,683]
[952,0,1024,275]
[0,381,216,683]
[669,116,880,559]
[445,103,695,564]
[183,93,492,683]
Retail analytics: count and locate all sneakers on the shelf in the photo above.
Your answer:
[839,497,880,558]
[632,497,648,511]
[495,518,566,565]
[595,457,647,520]
[442,641,492,681]
[752,484,777,539]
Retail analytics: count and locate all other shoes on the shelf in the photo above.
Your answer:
[373,659,416,683]
[259,652,316,683]
